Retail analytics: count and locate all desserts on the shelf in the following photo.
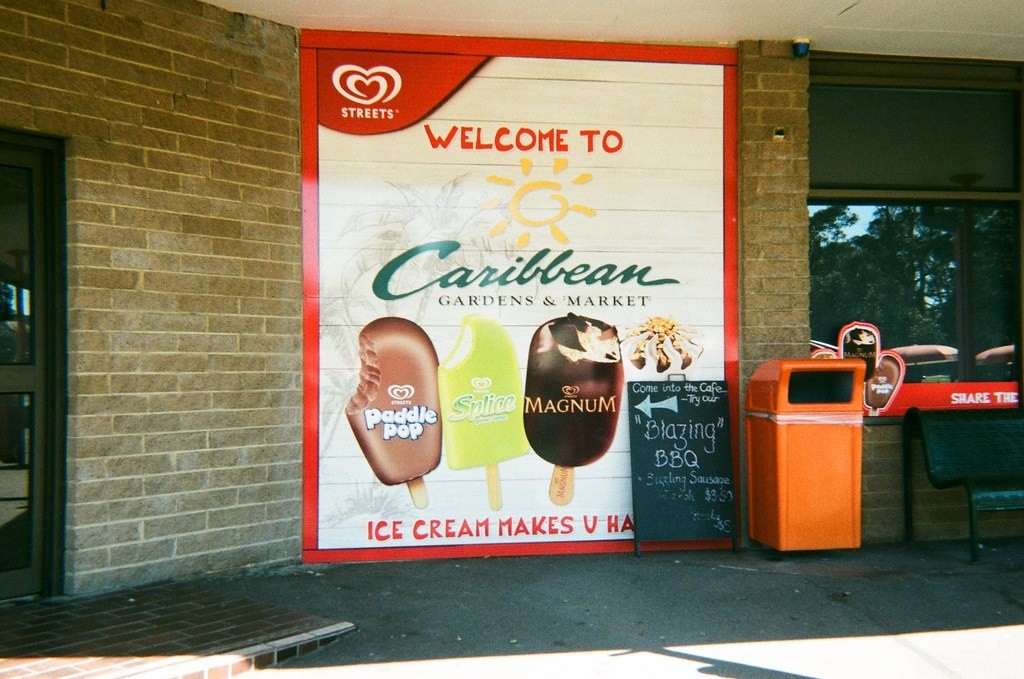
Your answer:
[625,317,705,380]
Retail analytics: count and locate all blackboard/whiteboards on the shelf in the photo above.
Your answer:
[625,378,739,542]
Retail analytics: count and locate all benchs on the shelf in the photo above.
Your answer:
[899,405,1024,562]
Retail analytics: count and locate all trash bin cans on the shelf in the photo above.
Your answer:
[745,355,868,553]
